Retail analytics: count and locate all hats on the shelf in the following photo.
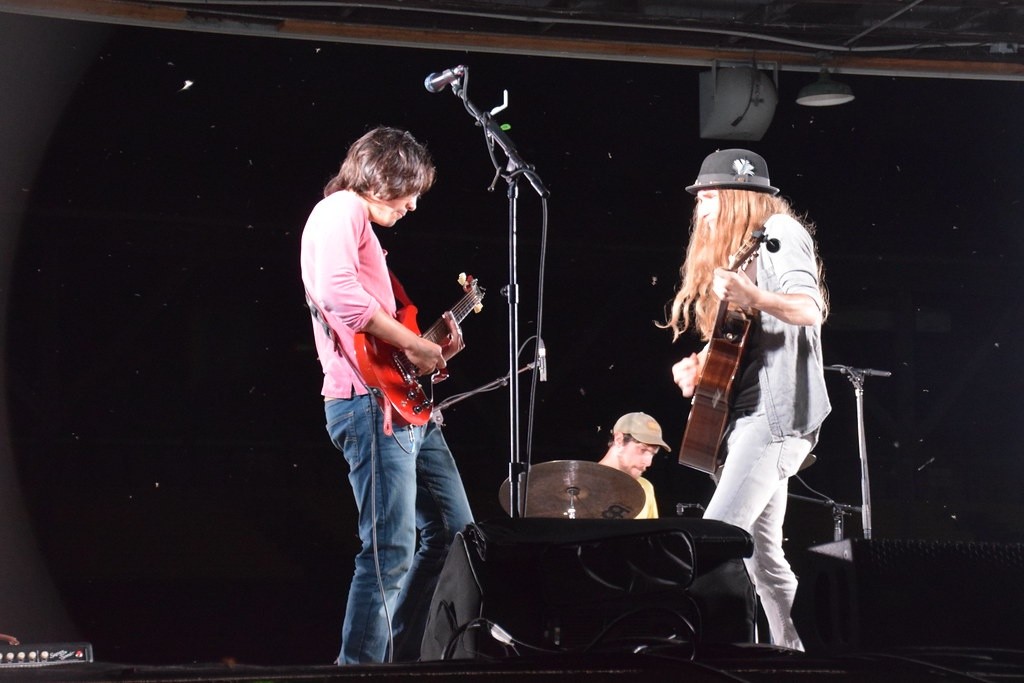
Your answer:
[685,148,780,196]
[611,411,671,453]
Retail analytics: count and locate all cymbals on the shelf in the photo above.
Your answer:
[501,461,645,519]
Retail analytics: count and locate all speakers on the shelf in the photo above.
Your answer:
[421,515,762,658]
[810,538,1023,657]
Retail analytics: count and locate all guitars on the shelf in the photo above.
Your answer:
[354,271,486,427]
[678,230,767,475]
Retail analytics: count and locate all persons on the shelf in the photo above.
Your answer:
[677,147,829,653]
[301,125,482,666]
[593,412,667,516]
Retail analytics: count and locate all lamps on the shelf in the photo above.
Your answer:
[793,60,856,106]
[700,56,783,143]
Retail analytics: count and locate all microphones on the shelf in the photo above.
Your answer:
[424,64,464,93]
